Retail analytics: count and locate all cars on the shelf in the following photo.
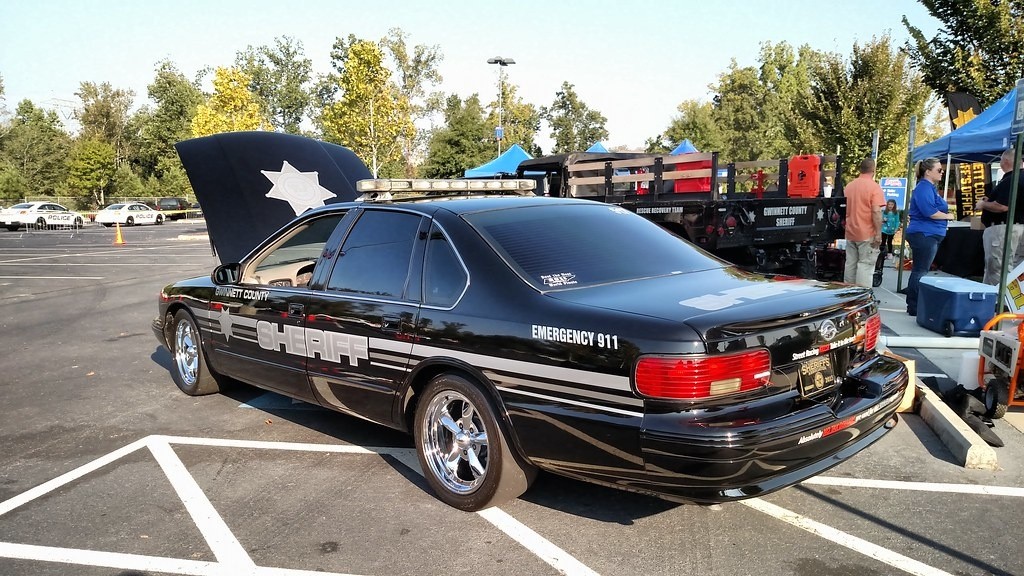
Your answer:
[95,203,166,227]
[0,201,84,230]
[156,196,191,220]
[153,131,907,512]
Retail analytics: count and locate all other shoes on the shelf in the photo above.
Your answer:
[907,307,917,315]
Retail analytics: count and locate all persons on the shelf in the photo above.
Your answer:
[843,158,888,289]
[904,157,954,316]
[879,199,900,260]
[976,148,1023,286]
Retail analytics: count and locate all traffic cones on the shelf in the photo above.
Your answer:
[112,222,125,245]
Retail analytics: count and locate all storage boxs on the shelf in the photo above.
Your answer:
[915,273,999,338]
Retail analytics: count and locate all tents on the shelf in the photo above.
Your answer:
[906,78,1023,222]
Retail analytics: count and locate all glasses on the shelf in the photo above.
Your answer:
[928,168,943,173]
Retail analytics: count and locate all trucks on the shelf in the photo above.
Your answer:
[494,154,847,278]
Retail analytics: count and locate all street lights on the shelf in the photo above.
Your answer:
[486,57,516,158]
